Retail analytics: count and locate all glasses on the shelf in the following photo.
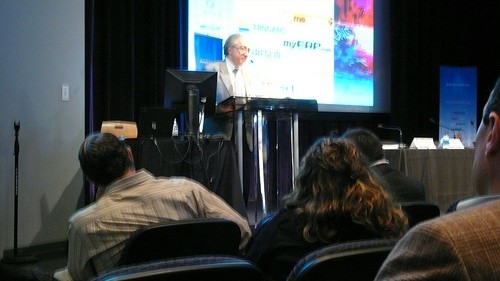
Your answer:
[232,46,249,53]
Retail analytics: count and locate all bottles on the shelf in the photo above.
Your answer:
[443,134,449,149]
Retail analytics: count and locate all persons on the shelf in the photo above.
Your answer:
[54,132,252,281]
[205,34,253,210]
[244,136,407,281]
[343,129,426,201]
[375,80,500,281]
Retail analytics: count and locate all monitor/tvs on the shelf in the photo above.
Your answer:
[163,69,217,139]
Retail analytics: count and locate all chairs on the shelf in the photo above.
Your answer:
[90,194,500,281]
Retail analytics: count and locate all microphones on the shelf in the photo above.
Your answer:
[378,124,403,149]
[430,119,456,138]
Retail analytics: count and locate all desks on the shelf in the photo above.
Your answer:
[383,149,474,210]
[130,139,247,219]
